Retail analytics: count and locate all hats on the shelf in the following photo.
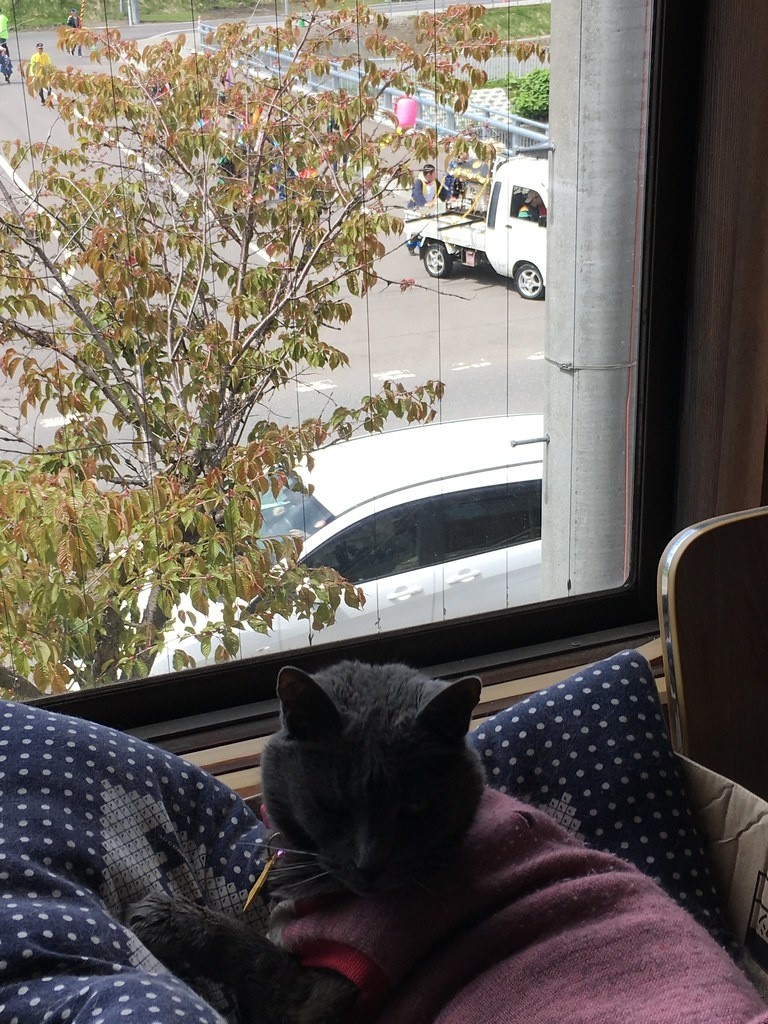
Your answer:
[524,190,539,203]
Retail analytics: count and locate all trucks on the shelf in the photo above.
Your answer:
[405,154,550,299]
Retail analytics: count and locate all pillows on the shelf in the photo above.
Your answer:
[0,698,273,1024]
[463,650,744,964]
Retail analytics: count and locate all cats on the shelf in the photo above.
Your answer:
[127,661,768,1024]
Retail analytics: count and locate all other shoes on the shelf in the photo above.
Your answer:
[409,248,414,256]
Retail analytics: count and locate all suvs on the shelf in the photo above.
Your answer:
[1,412,547,693]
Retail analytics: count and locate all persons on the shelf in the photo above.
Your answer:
[67,9,83,58]
[0,49,13,83]
[406,164,458,255]
[143,62,349,225]
[0,8,10,57]
[31,43,53,108]
[442,157,467,205]
[517,189,546,227]
